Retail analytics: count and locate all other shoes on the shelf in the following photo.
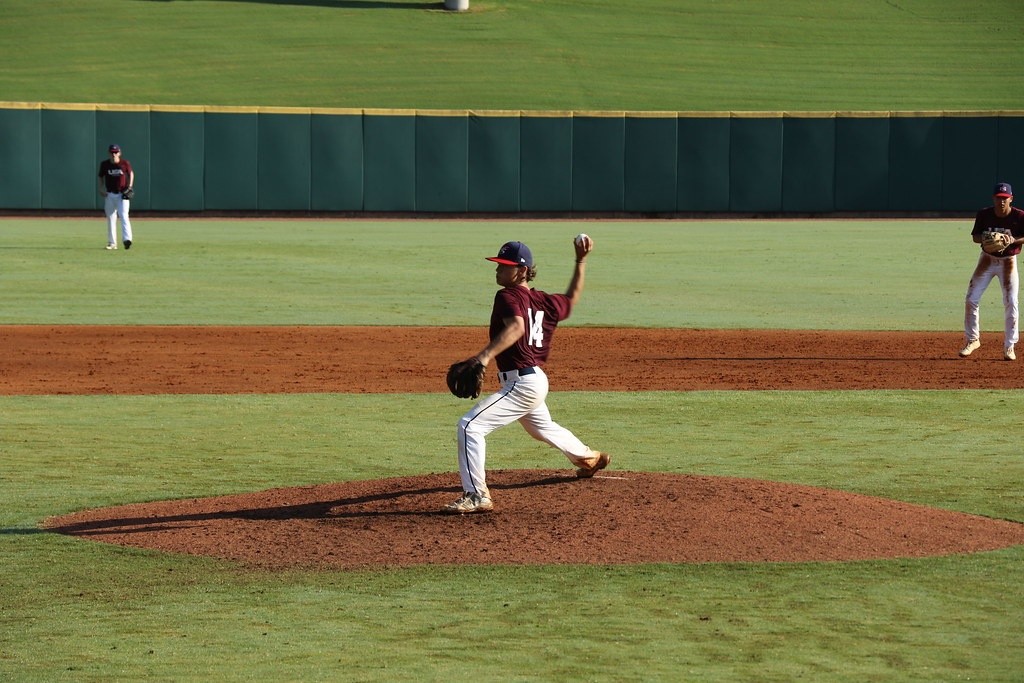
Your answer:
[105,244,118,250]
[124,240,132,249]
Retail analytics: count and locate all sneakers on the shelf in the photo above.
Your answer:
[578,453,611,479]
[959,338,980,356]
[1003,347,1016,360]
[444,492,494,513]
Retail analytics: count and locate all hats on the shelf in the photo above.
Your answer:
[109,144,121,153]
[993,183,1012,198]
[485,240,532,267]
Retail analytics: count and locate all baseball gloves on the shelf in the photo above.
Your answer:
[445,357,486,400]
[981,231,1014,254]
[121,187,134,199]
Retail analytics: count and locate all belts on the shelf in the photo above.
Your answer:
[107,189,123,194]
[499,367,535,383]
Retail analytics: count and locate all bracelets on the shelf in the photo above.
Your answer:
[576,261,585,263]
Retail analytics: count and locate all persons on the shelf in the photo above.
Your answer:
[98,144,134,250]
[958,183,1024,360]
[443,236,610,514]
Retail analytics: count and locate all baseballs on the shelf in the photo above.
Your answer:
[576,234,588,247]
